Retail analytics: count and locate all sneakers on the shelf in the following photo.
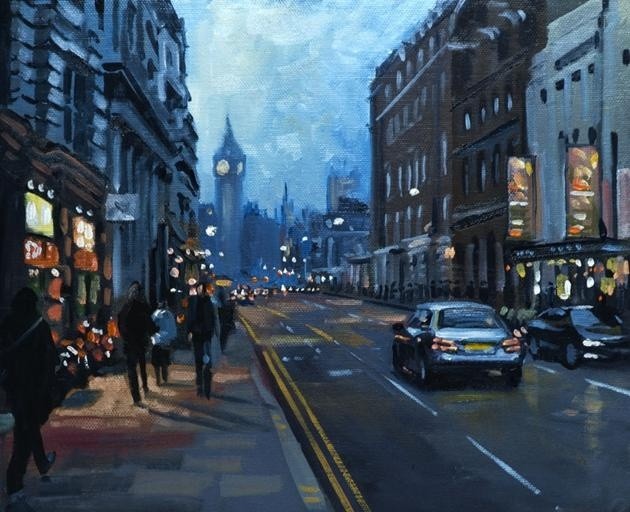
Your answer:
[39,450,57,475]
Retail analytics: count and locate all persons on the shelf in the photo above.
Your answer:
[0,285,66,512]
[328,278,558,309]
[115,280,238,405]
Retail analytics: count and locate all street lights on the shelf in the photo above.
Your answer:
[302,258,307,278]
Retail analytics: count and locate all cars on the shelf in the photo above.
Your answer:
[523,304,630,370]
[389,299,528,387]
